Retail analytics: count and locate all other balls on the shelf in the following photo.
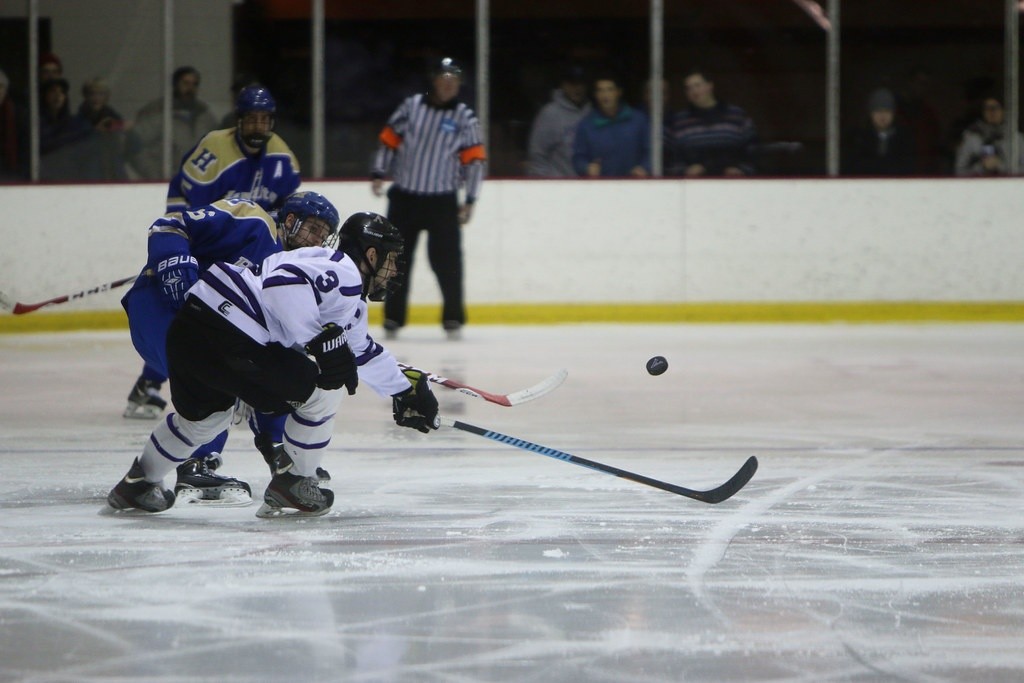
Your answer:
[646,356,669,377]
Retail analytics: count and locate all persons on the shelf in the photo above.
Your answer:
[121,86,301,418]
[527,63,759,177]
[0,54,263,179]
[954,95,1024,176]
[121,191,339,507]
[99,213,442,519]
[371,59,487,340]
[847,98,916,174]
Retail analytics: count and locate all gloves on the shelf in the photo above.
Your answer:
[392,370,442,434]
[155,255,199,311]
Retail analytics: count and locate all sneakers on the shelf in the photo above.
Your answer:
[383,318,401,341]
[174,451,254,507]
[122,377,167,419]
[257,467,335,517]
[98,456,175,516]
[443,318,461,342]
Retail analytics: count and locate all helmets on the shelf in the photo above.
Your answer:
[234,84,279,148]
[277,191,340,250]
[338,211,408,302]
[424,55,472,110]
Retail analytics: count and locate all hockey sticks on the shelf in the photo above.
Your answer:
[436,415,758,504]
[0,274,140,316]
[395,360,569,406]
